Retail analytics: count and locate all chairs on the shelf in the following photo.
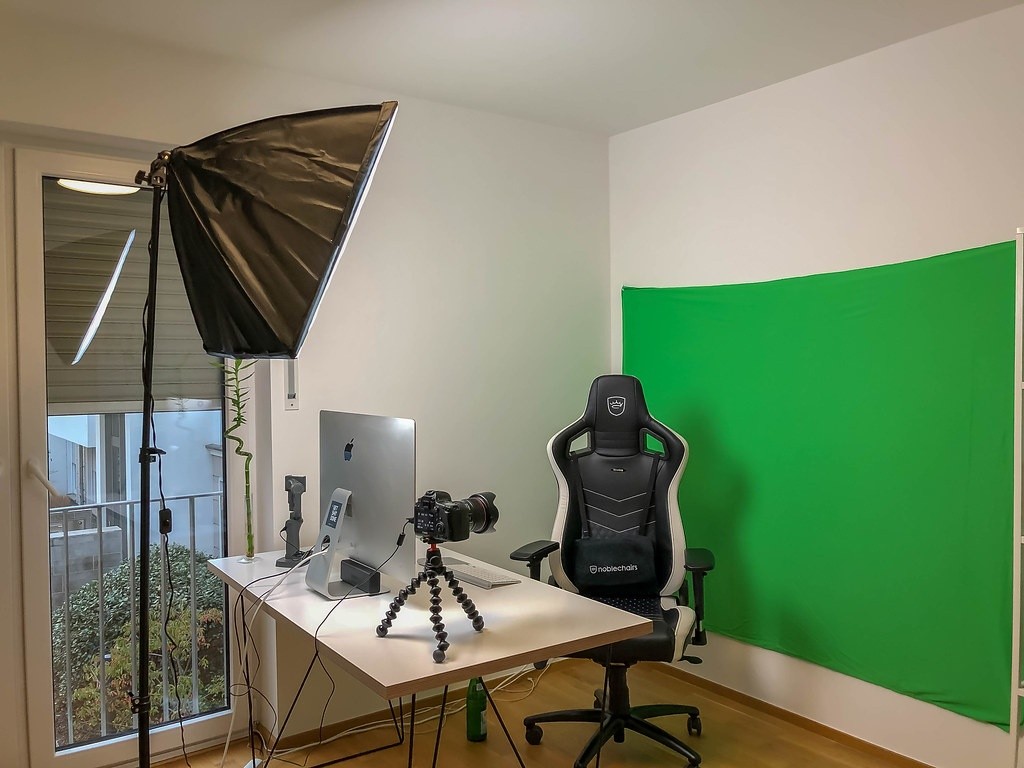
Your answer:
[510,376,715,768]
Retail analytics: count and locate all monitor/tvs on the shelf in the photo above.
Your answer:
[305,411,416,600]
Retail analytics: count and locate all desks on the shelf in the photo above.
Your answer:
[205,539,654,768]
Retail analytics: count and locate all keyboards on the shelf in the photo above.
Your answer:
[418,556,522,589]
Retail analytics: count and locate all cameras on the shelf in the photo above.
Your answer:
[413,490,499,545]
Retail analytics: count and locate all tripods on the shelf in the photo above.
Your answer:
[377,538,485,662]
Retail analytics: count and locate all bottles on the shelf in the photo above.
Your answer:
[466,677,487,742]
